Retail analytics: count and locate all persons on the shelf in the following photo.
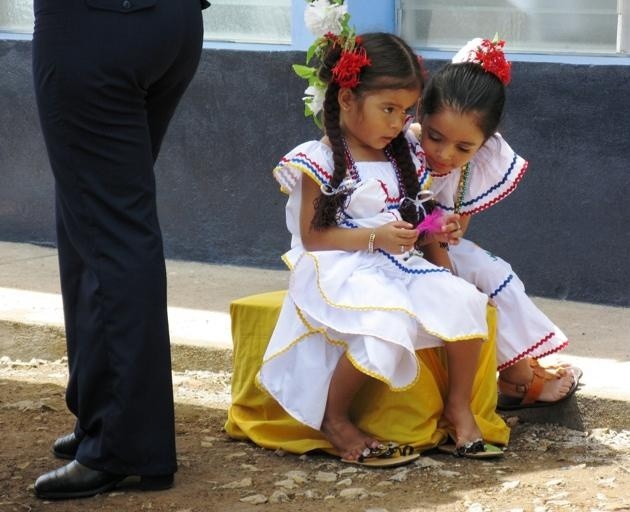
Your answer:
[255,32,504,470]
[409,39,583,412]
[31,0,210,500]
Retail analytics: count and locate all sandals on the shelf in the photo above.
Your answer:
[497,357,582,411]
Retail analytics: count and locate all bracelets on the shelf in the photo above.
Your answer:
[368,232,379,254]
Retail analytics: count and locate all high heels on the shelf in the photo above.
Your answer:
[33,431,176,500]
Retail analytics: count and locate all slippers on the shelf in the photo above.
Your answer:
[341,441,420,467]
[437,430,505,458]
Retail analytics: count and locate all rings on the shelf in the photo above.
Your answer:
[401,245,405,253]
[455,221,462,231]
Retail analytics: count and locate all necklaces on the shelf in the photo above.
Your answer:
[430,161,471,213]
[342,140,408,212]
